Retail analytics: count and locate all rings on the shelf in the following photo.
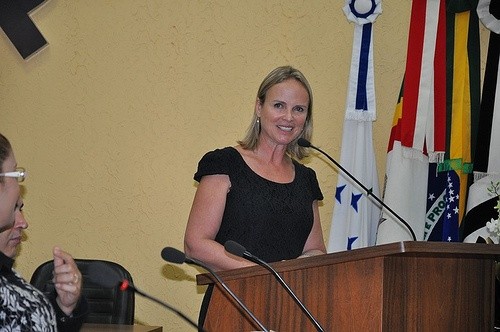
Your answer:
[74,273,78,284]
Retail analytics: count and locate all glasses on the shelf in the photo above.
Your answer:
[0,167,26,182]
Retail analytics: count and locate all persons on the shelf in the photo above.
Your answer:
[184,66,326,332]
[0,188,82,332]
[0,132,57,332]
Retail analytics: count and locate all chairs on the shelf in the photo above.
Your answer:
[30,259,135,326]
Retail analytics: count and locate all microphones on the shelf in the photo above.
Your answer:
[225,241,326,332]
[161,247,269,332]
[297,138,416,241]
[86,261,206,332]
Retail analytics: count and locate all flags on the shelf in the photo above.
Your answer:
[325,0,383,253]
[377,0,500,247]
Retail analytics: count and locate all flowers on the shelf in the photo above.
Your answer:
[487,180,500,244]
